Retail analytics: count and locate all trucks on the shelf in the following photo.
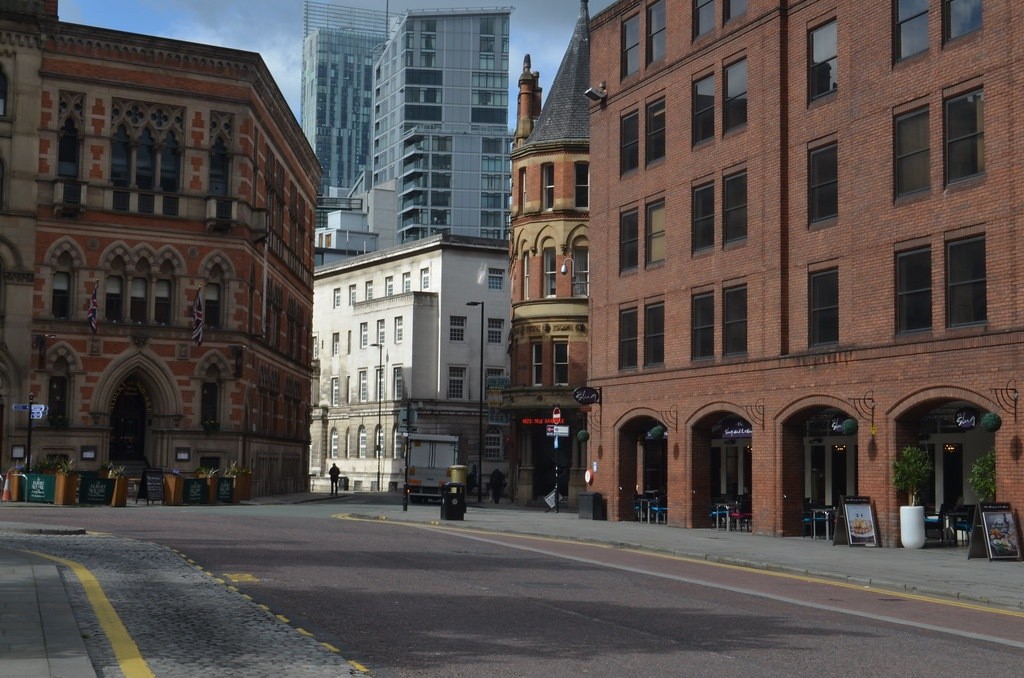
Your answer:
[405,433,459,503]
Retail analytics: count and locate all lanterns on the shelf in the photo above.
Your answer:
[981,413,1002,432]
[841,418,858,437]
[651,425,665,442]
[576,430,590,443]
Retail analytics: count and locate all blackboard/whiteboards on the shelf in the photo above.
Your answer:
[137,469,167,500]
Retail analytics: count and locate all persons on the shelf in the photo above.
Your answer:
[488,468,507,504]
[466,470,477,496]
[329,463,340,496]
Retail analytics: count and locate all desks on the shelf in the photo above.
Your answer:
[810,509,843,543]
[637,499,659,524]
[714,502,743,533]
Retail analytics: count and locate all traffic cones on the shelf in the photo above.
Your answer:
[1,473,13,502]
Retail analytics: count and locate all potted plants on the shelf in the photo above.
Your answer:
[892,446,933,549]
[10,455,253,507]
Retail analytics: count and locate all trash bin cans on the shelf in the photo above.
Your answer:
[441,483,466,520]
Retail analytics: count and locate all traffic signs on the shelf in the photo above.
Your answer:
[546,425,569,437]
[12,403,48,419]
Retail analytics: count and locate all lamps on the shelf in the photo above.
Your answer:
[582,81,607,101]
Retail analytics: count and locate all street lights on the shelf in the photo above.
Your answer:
[466,301,485,501]
[370,343,382,490]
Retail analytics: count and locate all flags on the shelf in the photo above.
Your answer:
[87,287,98,334]
[191,293,203,348]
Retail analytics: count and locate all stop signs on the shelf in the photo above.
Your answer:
[553,408,561,425]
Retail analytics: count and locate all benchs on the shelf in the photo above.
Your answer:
[634,506,972,547]
[409,493,442,506]
